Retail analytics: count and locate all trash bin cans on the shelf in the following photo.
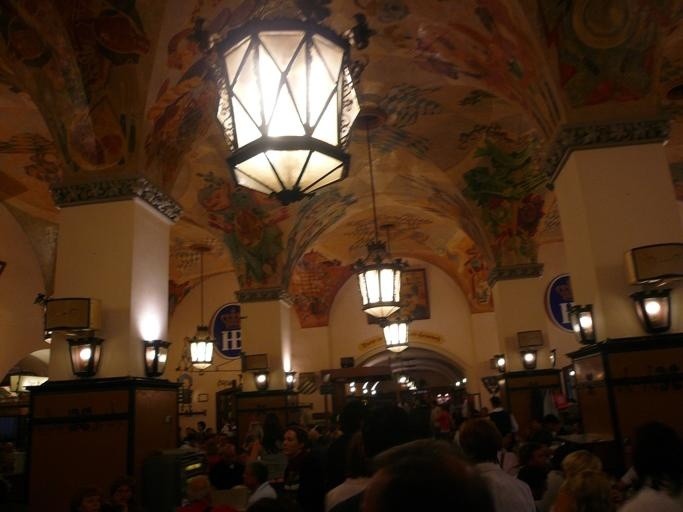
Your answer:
[152,447,208,511]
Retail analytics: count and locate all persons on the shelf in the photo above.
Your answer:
[68,396,683,512]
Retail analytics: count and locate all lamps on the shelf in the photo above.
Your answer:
[189,244,217,372]
[7,370,52,391]
[381,315,411,354]
[630,286,673,335]
[192,1,368,204]
[69,331,104,377]
[35,293,54,345]
[357,119,408,317]
[568,304,595,344]
[251,368,269,391]
[520,350,537,370]
[144,339,172,377]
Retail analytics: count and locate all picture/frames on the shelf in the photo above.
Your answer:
[367,268,430,323]
